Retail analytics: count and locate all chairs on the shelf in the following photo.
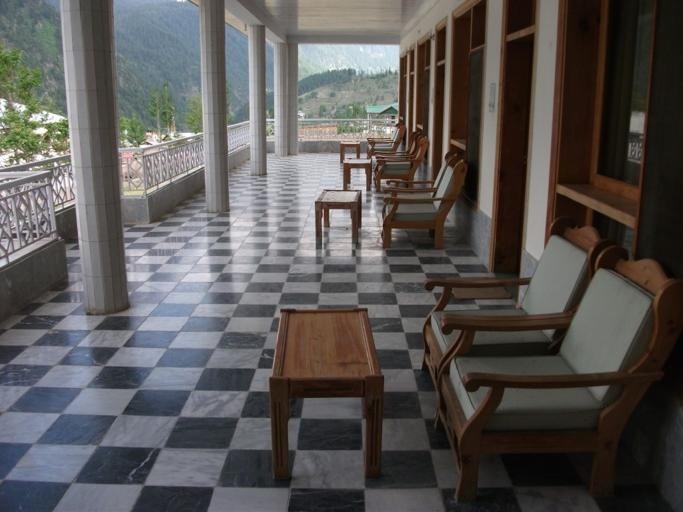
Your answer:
[423,246,682,504]
[423,217,621,432]
[367,122,468,250]
